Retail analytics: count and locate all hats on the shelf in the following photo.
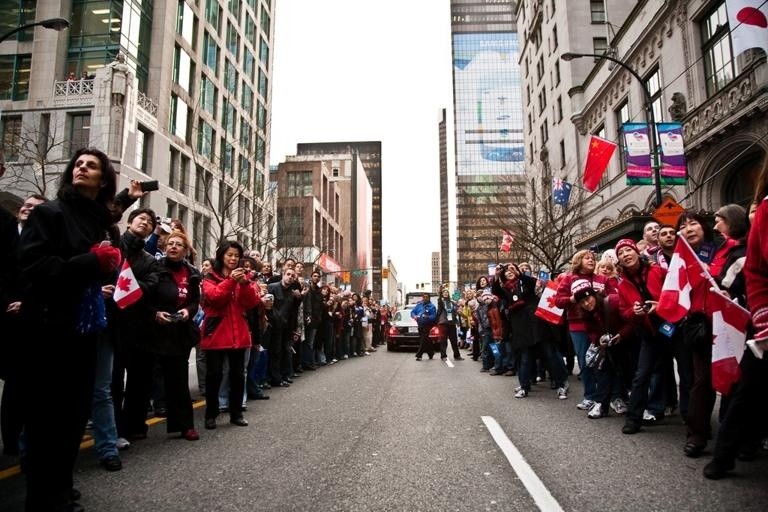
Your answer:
[616,239,640,257]
[571,276,593,303]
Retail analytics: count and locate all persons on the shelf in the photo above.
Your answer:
[555,160,768,479]
[412,262,568,399]
[196,242,392,429]
[1,148,198,512]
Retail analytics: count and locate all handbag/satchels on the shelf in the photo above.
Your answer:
[584,341,606,370]
[678,312,712,344]
[78,285,107,337]
[182,320,202,345]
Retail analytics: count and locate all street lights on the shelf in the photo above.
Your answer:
[559,52,666,213]
[472,235,499,265]
[1,15,71,41]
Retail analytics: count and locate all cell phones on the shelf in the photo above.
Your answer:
[238,259,245,268]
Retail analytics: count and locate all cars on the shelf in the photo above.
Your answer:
[384,304,449,353]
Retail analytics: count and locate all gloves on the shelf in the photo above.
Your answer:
[90,243,123,273]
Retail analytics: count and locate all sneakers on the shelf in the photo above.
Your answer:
[200,367,304,429]
[576,389,689,434]
[514,367,573,399]
[467,346,516,376]
[683,426,767,478]
[73,489,85,512]
[305,343,386,370]
[416,352,465,361]
[86,397,201,471]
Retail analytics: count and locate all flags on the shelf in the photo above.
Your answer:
[583,135,617,191]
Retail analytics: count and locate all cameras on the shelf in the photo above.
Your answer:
[156,216,171,225]
[498,264,508,272]
[166,313,184,322]
[600,332,613,346]
[636,303,652,313]
[140,181,160,192]
[255,271,263,277]
